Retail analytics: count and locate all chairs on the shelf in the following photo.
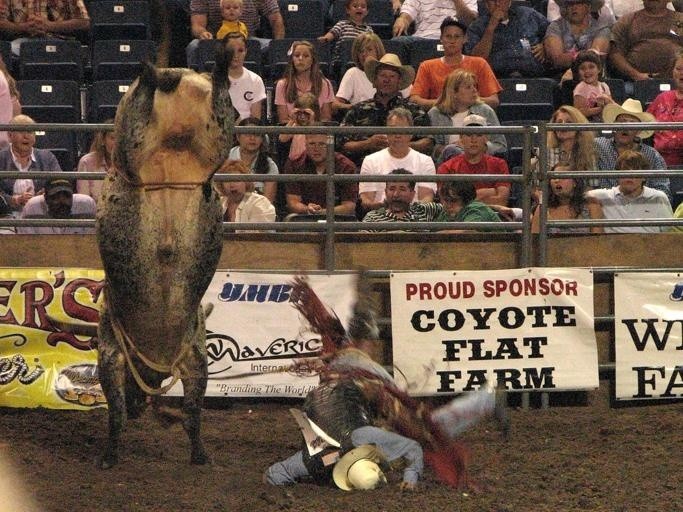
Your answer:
[18,0,677,233]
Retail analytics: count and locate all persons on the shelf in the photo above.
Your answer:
[0,0,92,59]
[75,120,116,204]
[0,114,62,234]
[18,177,101,235]
[263,265,510,493]
[0,56,22,148]
[186,2,682,235]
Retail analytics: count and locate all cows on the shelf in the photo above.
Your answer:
[95,38,236,469]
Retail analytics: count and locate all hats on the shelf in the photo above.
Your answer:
[555,0,606,11]
[462,115,486,126]
[333,444,386,492]
[602,98,655,138]
[364,53,416,89]
[45,177,72,196]
